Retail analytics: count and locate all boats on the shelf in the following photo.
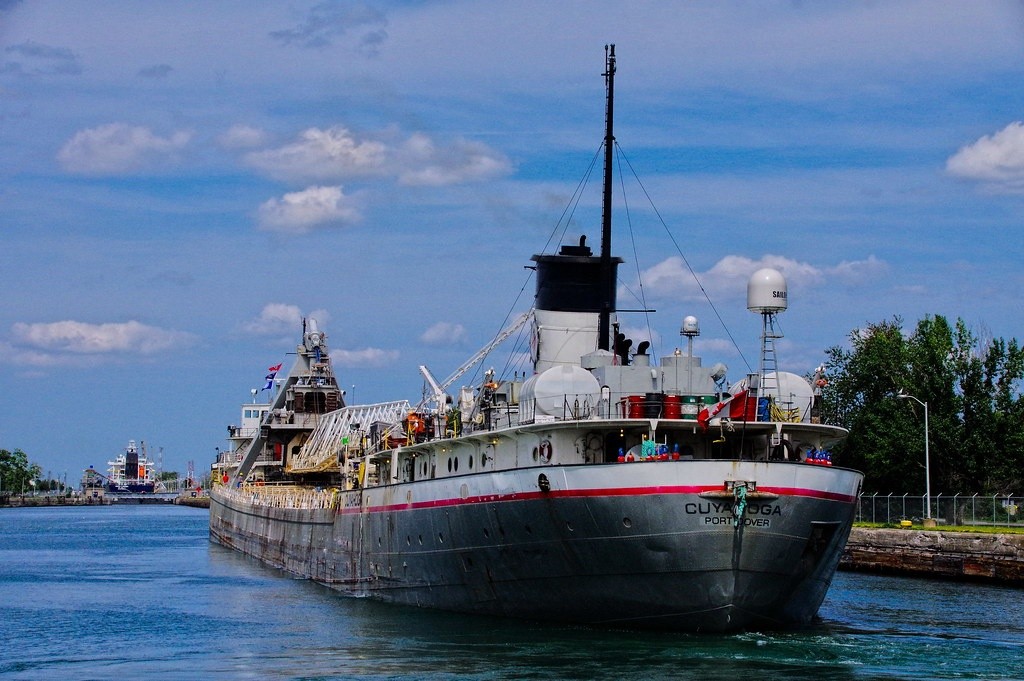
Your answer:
[208,42,865,635]
[104,440,179,504]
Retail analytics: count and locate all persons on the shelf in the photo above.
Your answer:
[237,472,244,489]
[352,476,360,491]
[223,473,228,482]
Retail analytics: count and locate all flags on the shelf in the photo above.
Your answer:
[699,391,745,433]
[262,363,282,391]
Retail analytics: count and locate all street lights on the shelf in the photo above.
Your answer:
[898,395,931,519]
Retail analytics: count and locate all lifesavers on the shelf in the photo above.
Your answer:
[539,439,552,464]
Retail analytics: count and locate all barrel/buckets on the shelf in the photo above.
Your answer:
[680,395,699,420]
[628,396,646,419]
[664,395,680,419]
[700,396,716,418]
[645,393,664,419]
[758,397,775,421]
